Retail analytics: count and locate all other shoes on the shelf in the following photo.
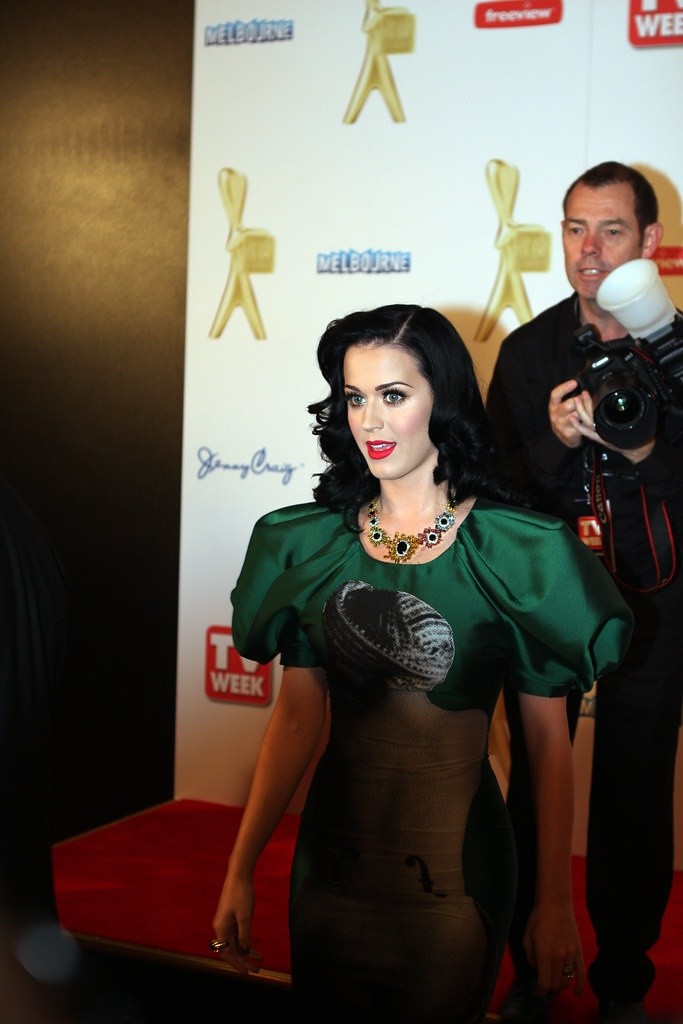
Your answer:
[598,1000,648,1024]
[501,981,556,1024]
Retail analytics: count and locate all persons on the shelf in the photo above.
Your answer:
[485,163,683,1024]
[213,304,635,1024]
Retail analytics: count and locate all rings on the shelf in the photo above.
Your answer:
[212,940,227,953]
[564,965,573,980]
[594,423,596,431]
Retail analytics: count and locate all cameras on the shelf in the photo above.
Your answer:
[557,257,683,450]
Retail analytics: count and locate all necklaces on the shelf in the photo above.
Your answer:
[368,494,457,560]
